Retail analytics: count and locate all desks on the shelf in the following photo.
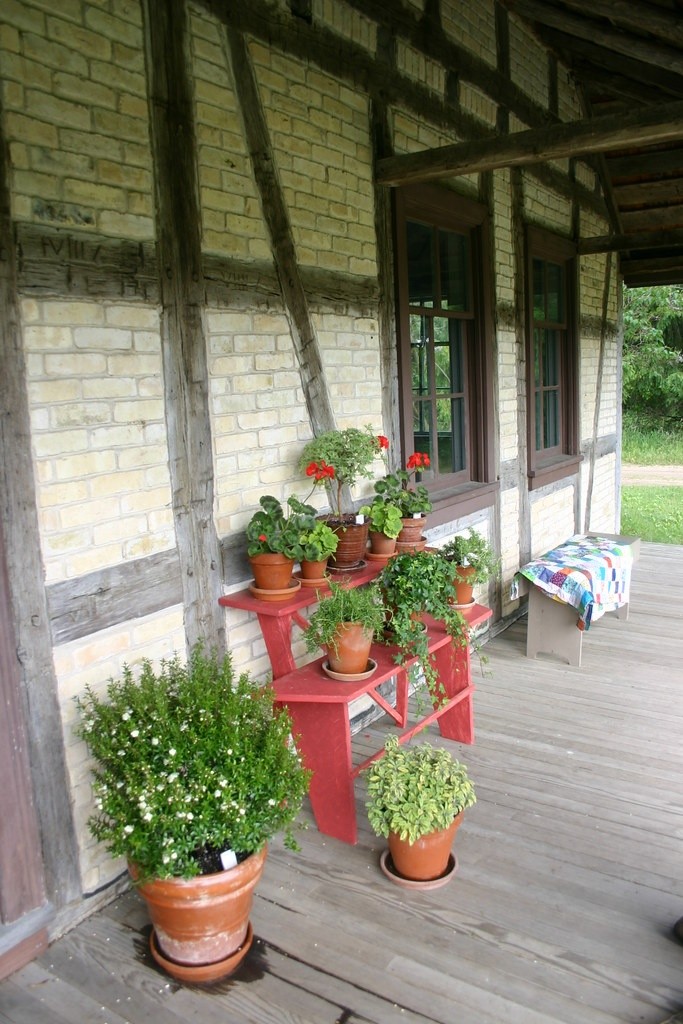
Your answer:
[510,530,642,666]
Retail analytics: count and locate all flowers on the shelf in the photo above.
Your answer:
[370,434,433,518]
[244,459,335,563]
[74,634,315,892]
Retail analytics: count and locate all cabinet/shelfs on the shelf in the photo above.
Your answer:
[217,547,494,847]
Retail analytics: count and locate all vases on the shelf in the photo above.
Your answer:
[394,536,427,554]
[127,839,268,966]
[396,513,427,542]
[246,554,295,590]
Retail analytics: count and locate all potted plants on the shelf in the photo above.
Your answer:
[438,526,503,605]
[299,520,338,579]
[297,428,383,569]
[377,547,492,718]
[298,569,395,674]
[358,731,477,880]
[358,501,406,555]
